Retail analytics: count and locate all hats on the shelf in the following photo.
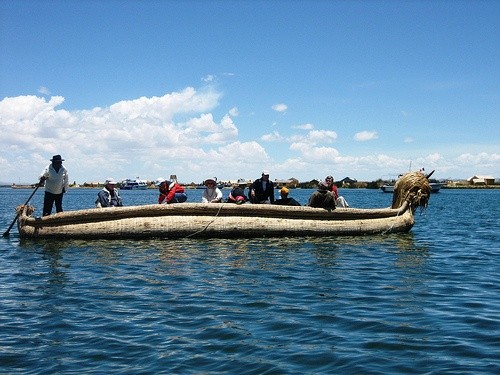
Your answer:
[262,170,269,176]
[155,177,166,186]
[278,186,289,194]
[104,177,118,185]
[237,178,248,185]
[204,177,216,182]
[50,155,65,162]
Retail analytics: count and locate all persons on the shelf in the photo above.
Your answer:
[304,179,337,210]
[39,155,68,216]
[201,176,223,202]
[95,178,124,209]
[227,179,249,204]
[326,176,351,208]
[156,177,187,204]
[248,171,275,204]
[275,186,301,205]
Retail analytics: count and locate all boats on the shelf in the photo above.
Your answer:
[15,171,432,239]
[381,162,447,194]
[195,180,224,189]
[117,177,148,189]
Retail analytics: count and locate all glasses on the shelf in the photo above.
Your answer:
[326,179,331,182]
